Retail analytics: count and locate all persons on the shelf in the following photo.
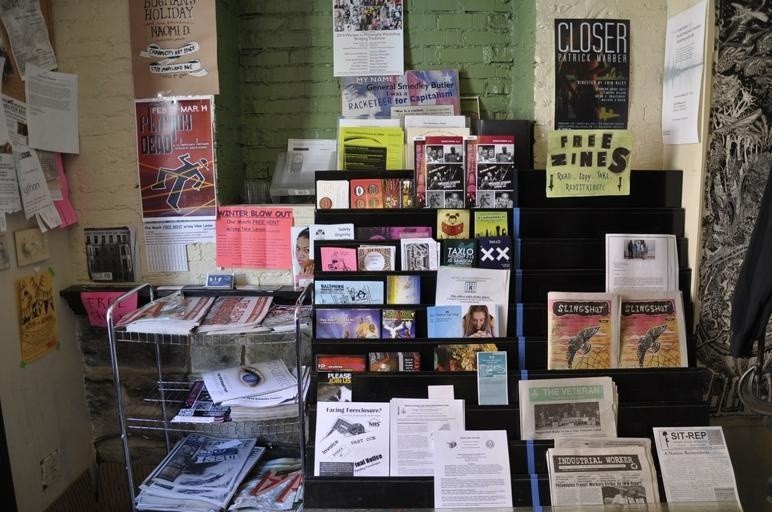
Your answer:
[295,228,315,279]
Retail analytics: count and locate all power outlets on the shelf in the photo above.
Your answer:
[14,228,51,267]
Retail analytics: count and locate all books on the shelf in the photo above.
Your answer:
[308,234,743,511]
[314,0,533,273]
[114,290,312,511]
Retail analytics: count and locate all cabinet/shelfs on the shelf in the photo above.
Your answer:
[304,170,710,512]
[106,283,315,512]
